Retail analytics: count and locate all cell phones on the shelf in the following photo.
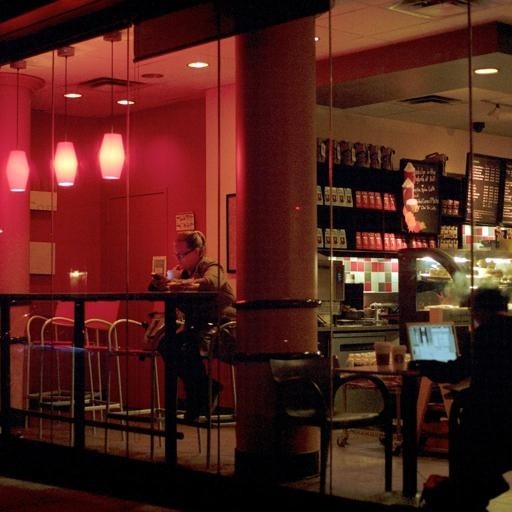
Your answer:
[151,272,161,280]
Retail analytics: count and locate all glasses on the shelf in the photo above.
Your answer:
[175,245,198,259]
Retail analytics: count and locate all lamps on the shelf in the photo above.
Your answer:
[4,31,126,192]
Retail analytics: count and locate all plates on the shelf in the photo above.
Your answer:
[165,284,200,290]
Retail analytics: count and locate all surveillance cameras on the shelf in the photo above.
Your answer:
[472,121,486,133]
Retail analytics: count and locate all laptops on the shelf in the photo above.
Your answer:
[406,320,462,363]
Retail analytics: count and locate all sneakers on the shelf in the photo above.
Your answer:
[183,377,224,424]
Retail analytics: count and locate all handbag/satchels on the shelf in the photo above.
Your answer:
[142,317,185,351]
[200,318,236,360]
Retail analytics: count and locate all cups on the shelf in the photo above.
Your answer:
[70,272,88,291]
[374,342,406,374]
[167,270,180,281]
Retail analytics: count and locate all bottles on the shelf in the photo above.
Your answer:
[429,307,442,323]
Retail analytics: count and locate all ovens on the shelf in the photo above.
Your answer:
[330,328,385,373]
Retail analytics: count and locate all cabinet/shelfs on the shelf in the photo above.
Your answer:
[315,160,465,259]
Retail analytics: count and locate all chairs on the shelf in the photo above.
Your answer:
[270,356,394,497]
[27,300,235,469]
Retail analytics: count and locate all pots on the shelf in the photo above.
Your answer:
[342,309,364,320]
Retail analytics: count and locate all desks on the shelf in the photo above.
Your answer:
[333,362,457,496]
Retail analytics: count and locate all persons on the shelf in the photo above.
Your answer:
[147,229,234,421]
[407,286,511,508]
